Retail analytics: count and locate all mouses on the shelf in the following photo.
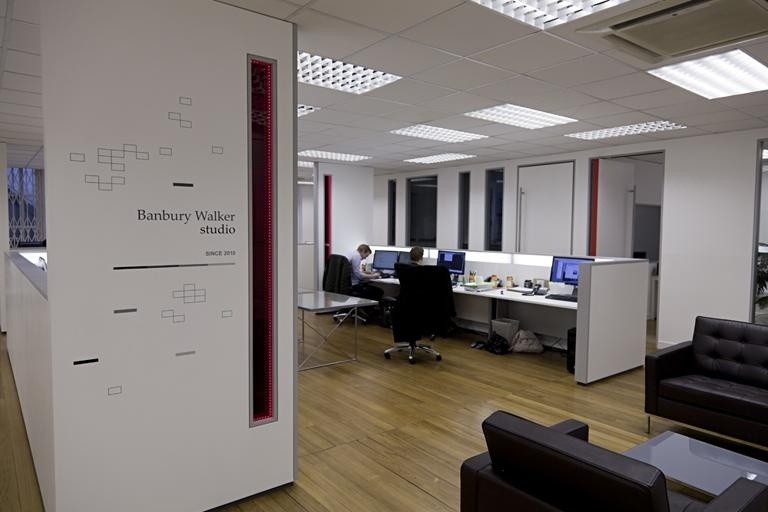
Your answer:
[452,281,457,285]
[383,274,390,278]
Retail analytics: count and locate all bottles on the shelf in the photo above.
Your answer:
[507,276,513,289]
[525,280,531,288]
[491,275,498,289]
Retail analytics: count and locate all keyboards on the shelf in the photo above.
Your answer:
[545,293,577,302]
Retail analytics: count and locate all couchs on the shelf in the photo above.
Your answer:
[646,315,768,448]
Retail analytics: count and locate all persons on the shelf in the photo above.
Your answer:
[407,246,424,266]
[346,243,383,325]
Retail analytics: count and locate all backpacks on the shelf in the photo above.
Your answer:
[511,328,544,354]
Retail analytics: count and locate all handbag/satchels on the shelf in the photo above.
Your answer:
[484,331,509,355]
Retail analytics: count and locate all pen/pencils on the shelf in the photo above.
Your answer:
[469,271,477,276]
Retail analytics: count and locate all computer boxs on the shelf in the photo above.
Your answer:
[382,296,398,328]
[566,327,576,375]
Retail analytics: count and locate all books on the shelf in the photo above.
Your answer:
[464,287,492,292]
[464,282,494,288]
[506,286,533,294]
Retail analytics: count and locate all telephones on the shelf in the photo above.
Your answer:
[533,284,549,295]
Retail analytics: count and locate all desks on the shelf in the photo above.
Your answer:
[297,287,380,370]
[368,270,578,348]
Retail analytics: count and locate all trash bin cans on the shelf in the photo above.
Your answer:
[491,318,520,346]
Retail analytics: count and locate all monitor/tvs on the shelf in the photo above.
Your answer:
[545,255,595,295]
[437,250,466,281]
[372,249,399,270]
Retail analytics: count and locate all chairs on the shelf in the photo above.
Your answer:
[460,410,768,509]
[384,266,449,364]
[327,254,373,326]
